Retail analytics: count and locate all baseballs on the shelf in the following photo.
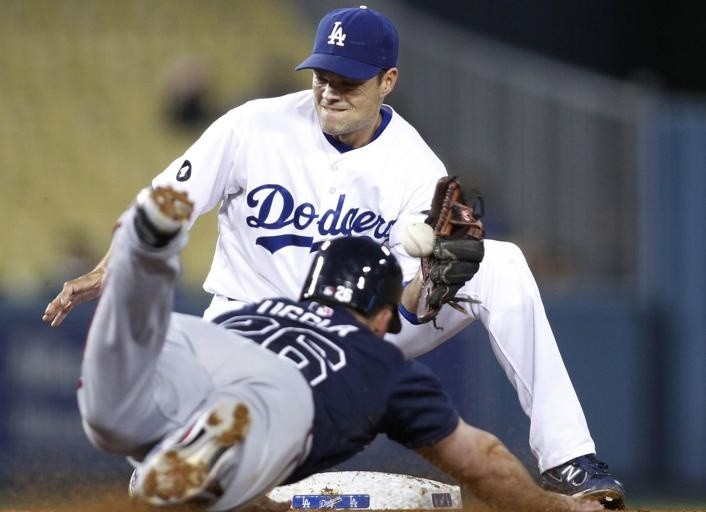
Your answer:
[403,222,436,257]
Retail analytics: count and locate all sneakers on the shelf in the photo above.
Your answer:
[129,398,251,508]
[539,454,626,509]
[133,184,194,248]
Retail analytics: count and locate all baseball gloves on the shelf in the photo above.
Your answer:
[417,175,485,324]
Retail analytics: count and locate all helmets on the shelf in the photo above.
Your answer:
[301,235,404,333]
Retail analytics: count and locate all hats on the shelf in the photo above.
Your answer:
[295,5,400,84]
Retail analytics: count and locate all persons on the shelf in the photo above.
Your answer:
[42,6,624,511]
[77,186,606,512]
